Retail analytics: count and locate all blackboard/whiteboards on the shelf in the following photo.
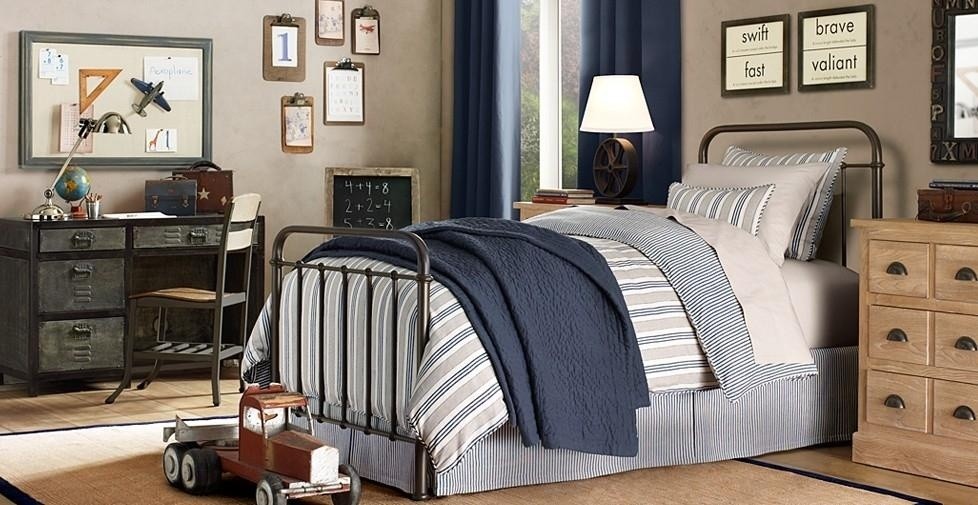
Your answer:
[324,168,420,242]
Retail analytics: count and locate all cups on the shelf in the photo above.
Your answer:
[86,201,100,219]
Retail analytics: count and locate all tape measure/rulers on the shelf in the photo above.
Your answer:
[79,69,122,114]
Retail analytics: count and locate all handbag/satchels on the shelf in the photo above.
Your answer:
[173,160,233,212]
[145,177,198,216]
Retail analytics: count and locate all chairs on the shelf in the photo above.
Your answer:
[106,193,261,402]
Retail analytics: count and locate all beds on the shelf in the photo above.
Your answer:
[239,123,884,499]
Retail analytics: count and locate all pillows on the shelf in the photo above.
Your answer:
[681,163,829,262]
[664,182,773,235]
[718,146,844,261]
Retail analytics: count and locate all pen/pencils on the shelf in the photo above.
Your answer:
[85,192,103,202]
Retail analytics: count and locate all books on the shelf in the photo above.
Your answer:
[101,211,176,220]
[534,188,594,198]
[532,197,594,205]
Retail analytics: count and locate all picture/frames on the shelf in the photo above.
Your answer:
[797,4,876,90]
[721,13,790,97]
[928,1,978,165]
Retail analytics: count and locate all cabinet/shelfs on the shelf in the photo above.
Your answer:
[845,216,977,488]
[509,200,594,223]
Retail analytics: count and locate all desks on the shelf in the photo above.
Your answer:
[0,211,265,395]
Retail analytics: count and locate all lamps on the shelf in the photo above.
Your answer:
[30,111,136,221]
[579,74,655,201]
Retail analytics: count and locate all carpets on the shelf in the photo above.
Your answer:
[0,411,939,505]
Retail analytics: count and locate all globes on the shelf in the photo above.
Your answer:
[56,167,90,216]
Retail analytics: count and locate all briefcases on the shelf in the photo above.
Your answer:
[917,188,978,223]
